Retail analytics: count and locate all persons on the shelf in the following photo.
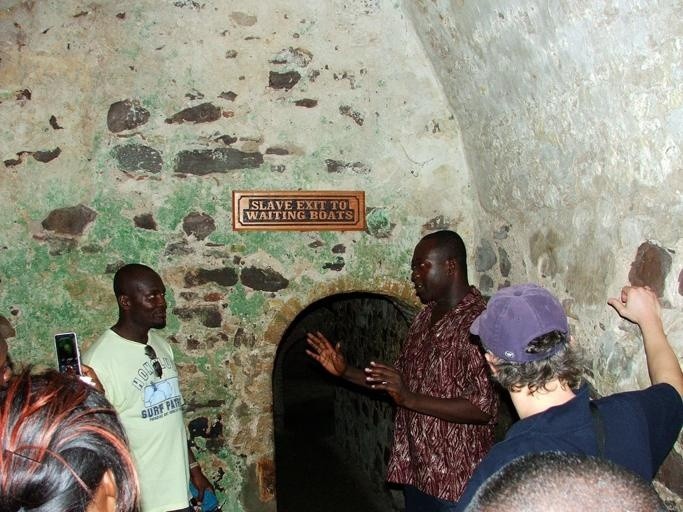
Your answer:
[456,448,667,512]
[82,260,215,512]
[303,229,500,512]
[0,359,141,512]
[453,282,681,512]
[0,328,106,396]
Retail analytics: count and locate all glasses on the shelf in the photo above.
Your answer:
[145,345,162,378]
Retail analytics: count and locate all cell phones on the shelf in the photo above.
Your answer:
[53,332,82,378]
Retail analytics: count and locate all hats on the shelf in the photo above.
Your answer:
[470,285,569,361]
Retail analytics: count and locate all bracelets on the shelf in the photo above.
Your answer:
[188,460,200,470]
